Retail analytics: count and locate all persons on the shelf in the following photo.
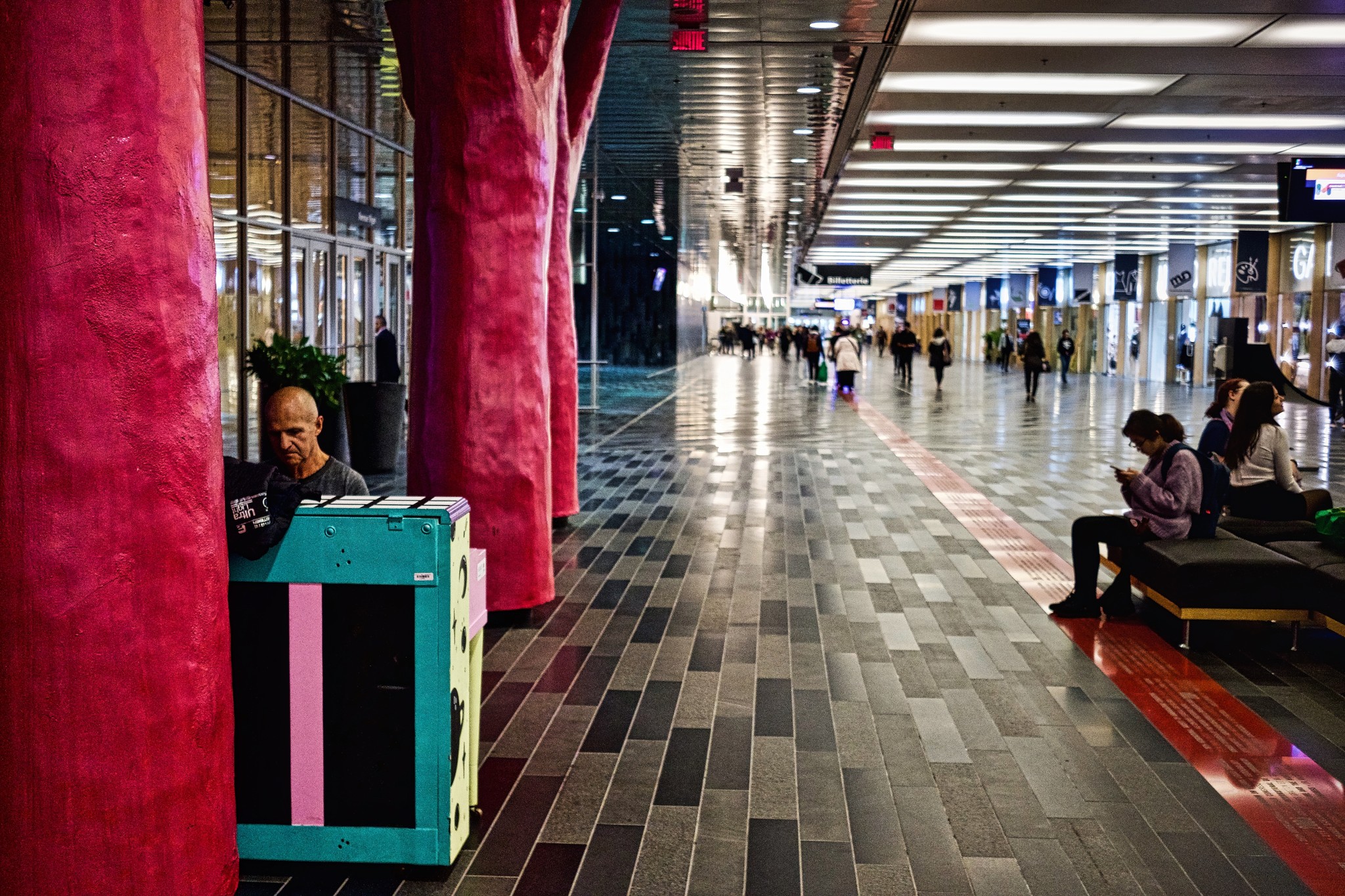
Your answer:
[717,318,1077,404]
[1223,380,1334,520]
[1195,376,1303,506]
[1327,314,1345,428]
[1050,409,1203,619]
[246,387,371,521]
[375,316,408,415]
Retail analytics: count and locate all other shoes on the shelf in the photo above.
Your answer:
[1180,379,1188,386]
[1330,406,1345,429]
[1048,589,1101,616]
[1026,393,1036,403]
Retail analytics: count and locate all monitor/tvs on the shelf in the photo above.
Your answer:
[1286,157,1345,214]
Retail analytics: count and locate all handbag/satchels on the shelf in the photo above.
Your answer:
[943,338,951,366]
[818,360,827,382]
[1039,358,1050,374]
[1314,506,1345,550]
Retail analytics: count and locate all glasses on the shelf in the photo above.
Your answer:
[1129,438,1147,449]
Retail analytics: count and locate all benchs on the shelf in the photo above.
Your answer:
[1214,510,1320,546]
[1264,539,1345,642]
[1097,524,1310,654]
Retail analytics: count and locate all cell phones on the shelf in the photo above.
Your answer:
[1109,465,1127,472]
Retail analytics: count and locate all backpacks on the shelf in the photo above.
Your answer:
[1146,442,1230,540]
[807,334,820,353]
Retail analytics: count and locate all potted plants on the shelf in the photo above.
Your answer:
[241,331,349,460]
[991,327,1005,363]
[980,331,994,363]
